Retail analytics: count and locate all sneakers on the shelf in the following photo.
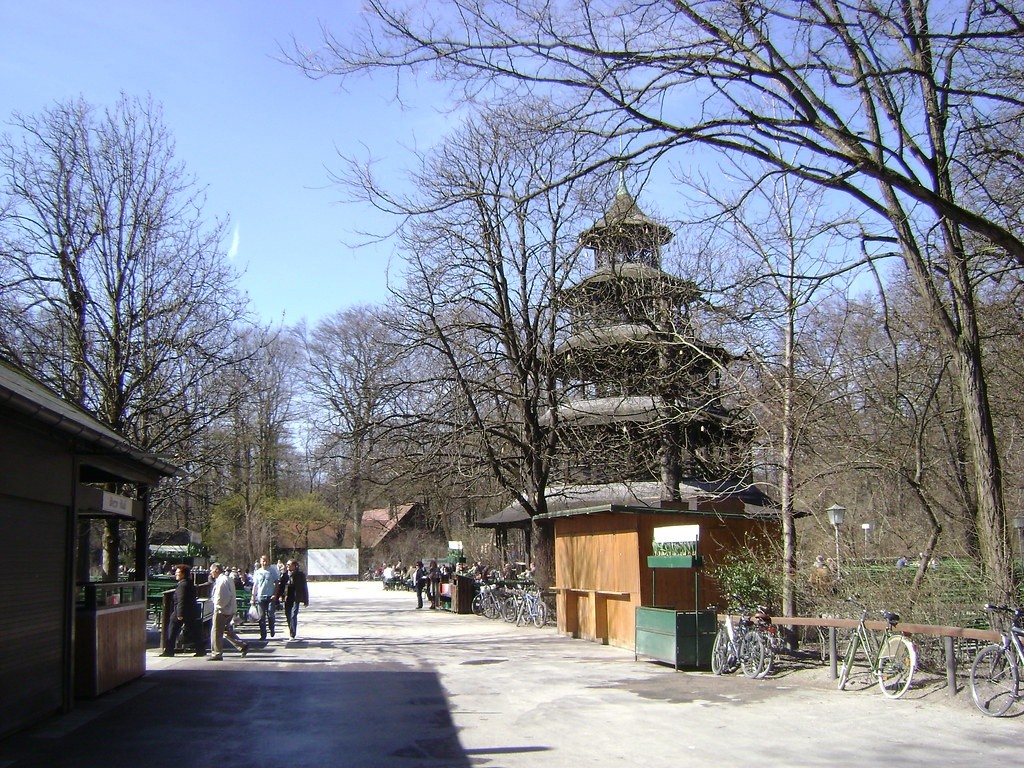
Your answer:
[289,636,294,640]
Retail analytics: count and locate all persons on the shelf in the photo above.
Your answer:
[250,555,280,640]
[278,559,310,642]
[118,558,299,592]
[158,564,207,657]
[206,562,251,663]
[365,555,517,613]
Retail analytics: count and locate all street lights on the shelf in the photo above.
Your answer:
[825,501,847,582]
[1014,514,1024,564]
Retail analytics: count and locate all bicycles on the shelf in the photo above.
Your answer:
[470,576,549,630]
[969,603,1024,718]
[730,596,778,679]
[706,596,766,679]
[836,592,920,700]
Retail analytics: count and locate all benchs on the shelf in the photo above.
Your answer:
[384,573,529,602]
[78,573,260,629]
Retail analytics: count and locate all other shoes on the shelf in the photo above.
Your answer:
[206,652,223,661]
[259,635,267,640]
[241,645,249,658]
[159,650,174,657]
[194,651,207,657]
[270,631,275,636]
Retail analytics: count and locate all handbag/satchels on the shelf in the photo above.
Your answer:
[248,604,259,621]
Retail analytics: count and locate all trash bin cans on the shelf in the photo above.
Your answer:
[449,574,475,614]
